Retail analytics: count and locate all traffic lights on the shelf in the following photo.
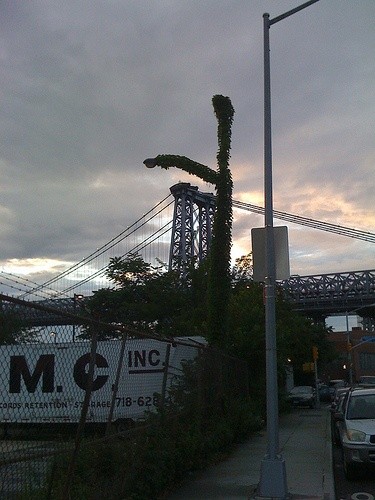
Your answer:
[348,342,352,352]
[312,346,318,360]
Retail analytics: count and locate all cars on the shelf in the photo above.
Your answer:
[311,372,375,447]
[287,386,316,408]
[335,387,375,479]
